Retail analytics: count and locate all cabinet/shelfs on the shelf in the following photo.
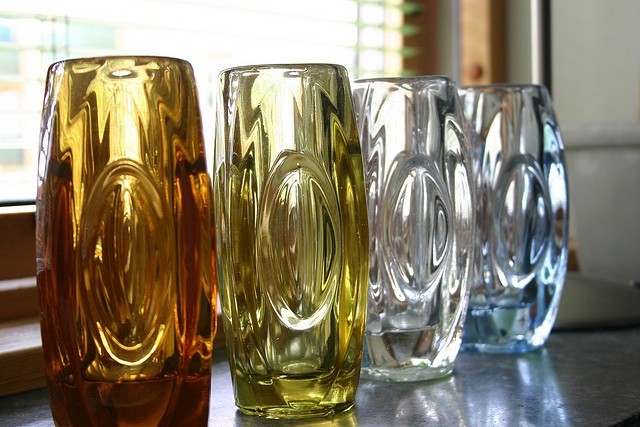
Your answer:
[1,205,225,396]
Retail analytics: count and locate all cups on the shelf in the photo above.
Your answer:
[35,55,216,427]
[216,62,369,422]
[455,84,570,355]
[359,75,475,382]
